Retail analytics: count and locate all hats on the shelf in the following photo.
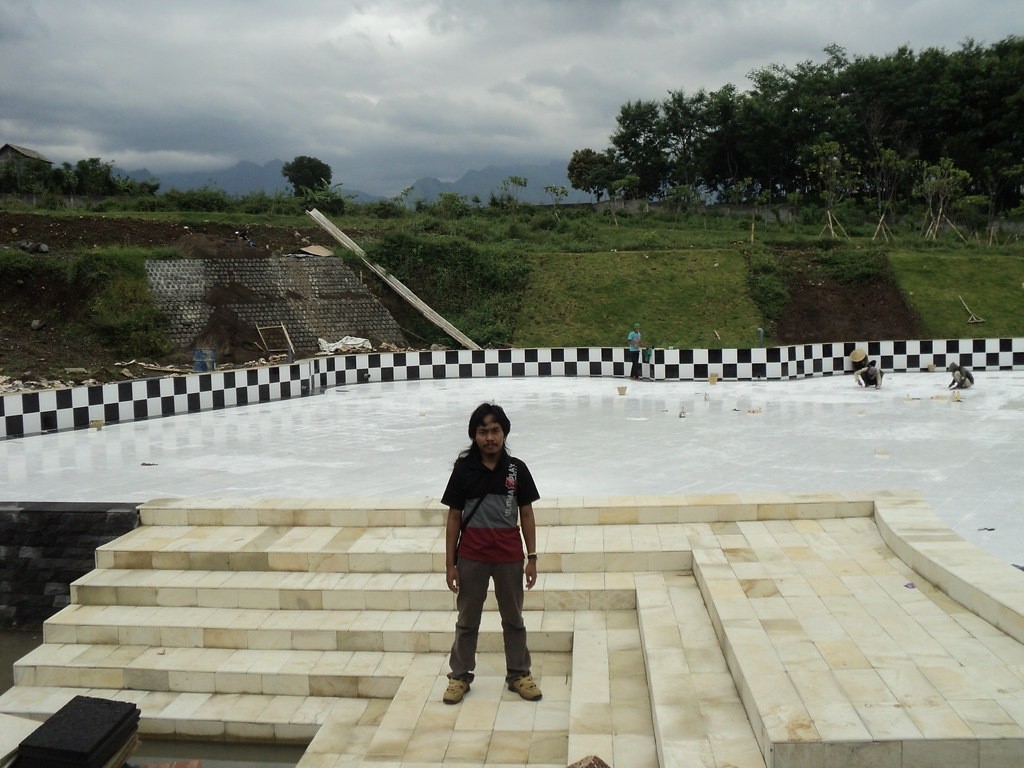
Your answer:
[633,323,642,330]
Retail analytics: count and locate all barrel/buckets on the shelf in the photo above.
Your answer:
[90,419,103,430]
[710,373,719,381]
[709,377,717,385]
[618,387,626,395]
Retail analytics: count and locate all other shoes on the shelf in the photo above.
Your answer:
[508,673,543,701]
[443,677,471,705]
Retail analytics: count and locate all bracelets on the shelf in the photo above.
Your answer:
[527,553,537,555]
[528,554,538,559]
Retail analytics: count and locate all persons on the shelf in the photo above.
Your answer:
[948,362,974,389]
[850,348,884,389]
[441,403,542,705]
[628,323,649,381]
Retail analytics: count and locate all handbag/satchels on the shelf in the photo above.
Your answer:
[453,547,459,566]
